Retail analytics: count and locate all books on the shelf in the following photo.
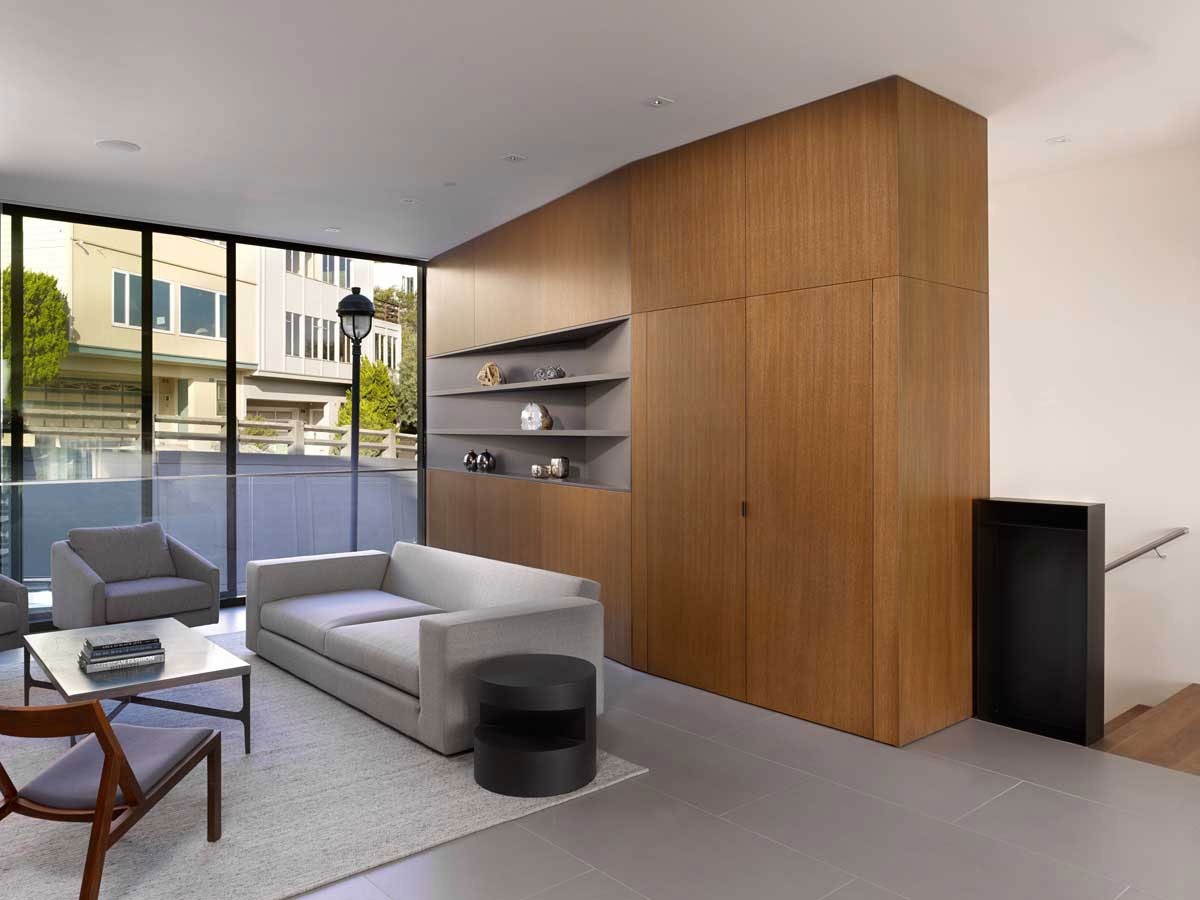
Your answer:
[76,629,166,673]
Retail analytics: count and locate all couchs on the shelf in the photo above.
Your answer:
[243,541,606,759]
[0,573,29,653]
[50,520,222,632]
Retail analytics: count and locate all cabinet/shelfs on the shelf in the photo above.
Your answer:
[426,312,634,492]
[633,277,991,750]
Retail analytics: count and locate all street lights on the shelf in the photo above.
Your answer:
[336,286,375,553]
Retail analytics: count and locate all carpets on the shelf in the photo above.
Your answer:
[0,627,652,900]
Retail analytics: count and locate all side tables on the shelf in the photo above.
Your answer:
[472,651,597,799]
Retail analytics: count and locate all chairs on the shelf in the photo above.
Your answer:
[0,698,223,900]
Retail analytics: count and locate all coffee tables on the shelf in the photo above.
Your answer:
[20,617,253,756]
